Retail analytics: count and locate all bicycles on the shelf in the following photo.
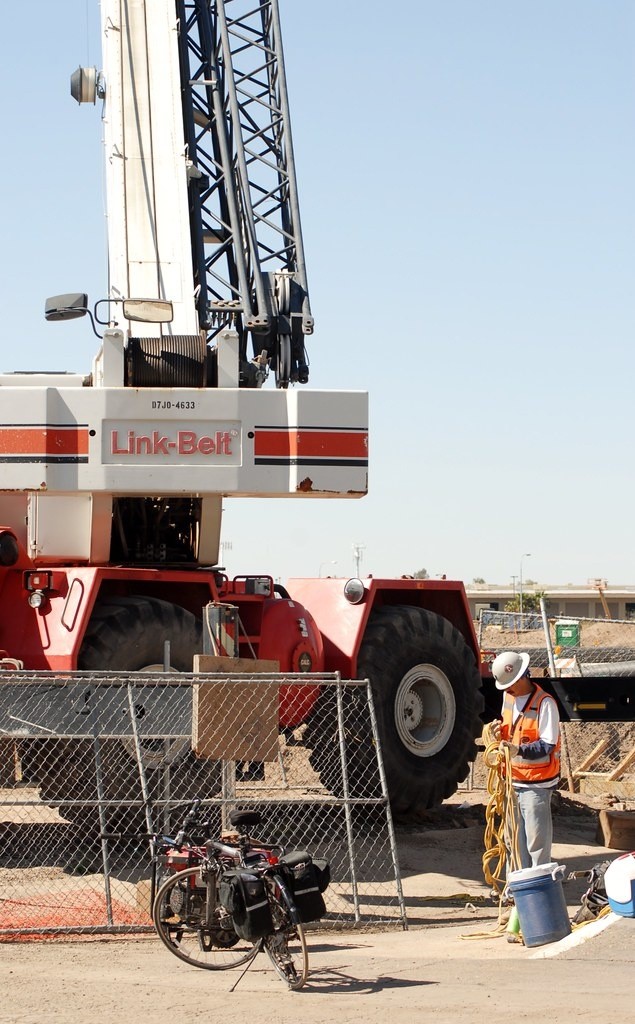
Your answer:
[151,798,308,993]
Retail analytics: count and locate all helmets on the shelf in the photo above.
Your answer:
[492,651,530,691]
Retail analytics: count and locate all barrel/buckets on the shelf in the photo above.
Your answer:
[509,862,571,948]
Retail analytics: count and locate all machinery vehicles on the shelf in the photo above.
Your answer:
[1,0,485,836]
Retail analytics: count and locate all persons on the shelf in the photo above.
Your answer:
[484,651,561,883]
